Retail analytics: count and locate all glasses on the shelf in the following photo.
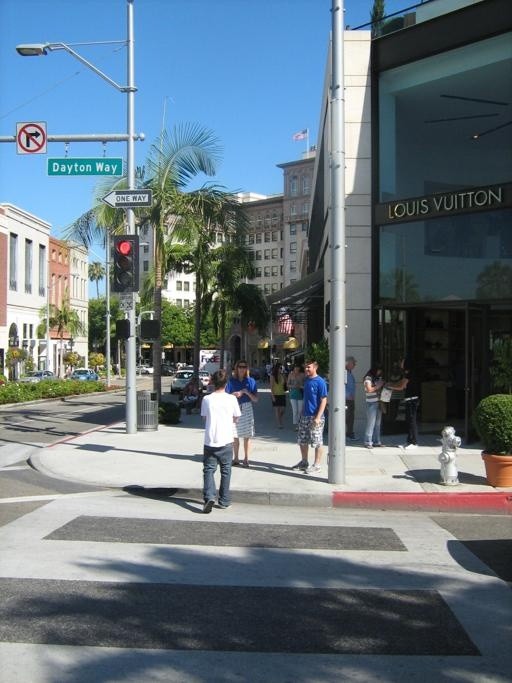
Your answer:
[237,365,247,368]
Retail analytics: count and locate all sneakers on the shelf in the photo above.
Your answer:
[347,432,360,441]
[203,499,215,513]
[232,459,250,467]
[291,461,323,475]
[365,444,386,449]
[398,442,418,449]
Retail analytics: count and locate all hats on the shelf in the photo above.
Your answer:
[346,356,357,362]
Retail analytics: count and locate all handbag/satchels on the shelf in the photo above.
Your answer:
[380,387,393,402]
[380,402,386,414]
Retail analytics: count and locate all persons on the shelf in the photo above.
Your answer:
[359,363,387,449]
[269,361,288,430]
[224,359,259,470]
[198,366,244,516]
[345,355,361,442]
[290,358,329,476]
[286,363,305,432]
[177,373,208,410]
[385,355,423,450]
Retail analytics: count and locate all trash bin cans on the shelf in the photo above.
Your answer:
[137,390,158,432]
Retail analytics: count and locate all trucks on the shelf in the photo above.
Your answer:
[197,347,233,386]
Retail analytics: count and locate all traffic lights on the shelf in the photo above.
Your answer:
[141,318,164,343]
[113,234,139,294]
[114,319,129,339]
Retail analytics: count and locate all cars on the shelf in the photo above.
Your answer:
[70,368,101,384]
[135,360,264,394]
[16,370,59,387]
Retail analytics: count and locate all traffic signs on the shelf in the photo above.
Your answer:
[47,157,124,177]
[101,189,153,208]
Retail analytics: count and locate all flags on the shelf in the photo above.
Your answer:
[291,128,307,141]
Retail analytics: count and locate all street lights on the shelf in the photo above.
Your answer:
[15,37,148,430]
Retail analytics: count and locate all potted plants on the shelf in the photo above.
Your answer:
[473,394,512,489]
[158,401,181,424]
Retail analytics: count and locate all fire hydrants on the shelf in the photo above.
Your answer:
[437,421,462,486]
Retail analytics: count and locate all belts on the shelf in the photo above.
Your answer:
[405,396,419,402]
